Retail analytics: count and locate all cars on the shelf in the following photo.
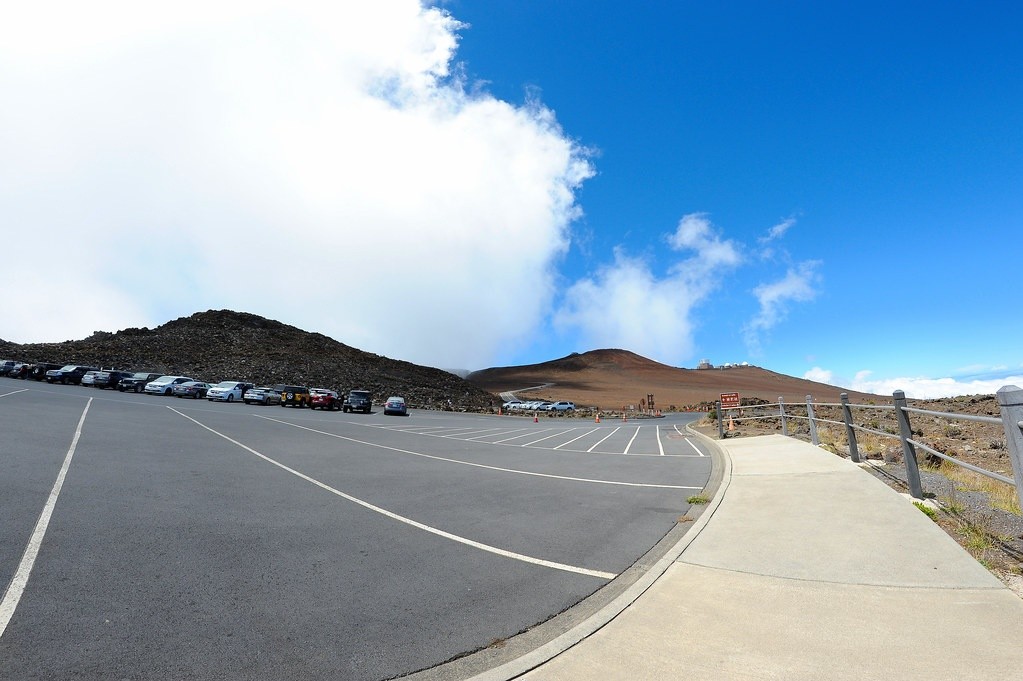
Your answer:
[383,396,407,416]
[533,401,554,410]
[172,381,212,399]
[144,376,195,396]
[204,381,247,403]
[81,370,100,387]
[503,400,542,410]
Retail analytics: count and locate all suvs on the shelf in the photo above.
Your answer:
[45,365,100,385]
[95,370,132,389]
[310,390,343,411]
[0,359,35,380]
[280,385,310,408]
[546,400,576,411]
[343,389,373,414]
[117,371,167,393]
[30,361,66,382]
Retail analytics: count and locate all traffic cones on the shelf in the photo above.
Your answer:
[622,413,627,422]
[595,414,600,423]
[533,412,538,423]
[655,410,661,416]
[498,406,503,415]
[728,414,735,431]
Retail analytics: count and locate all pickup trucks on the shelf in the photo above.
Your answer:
[244,387,282,406]
